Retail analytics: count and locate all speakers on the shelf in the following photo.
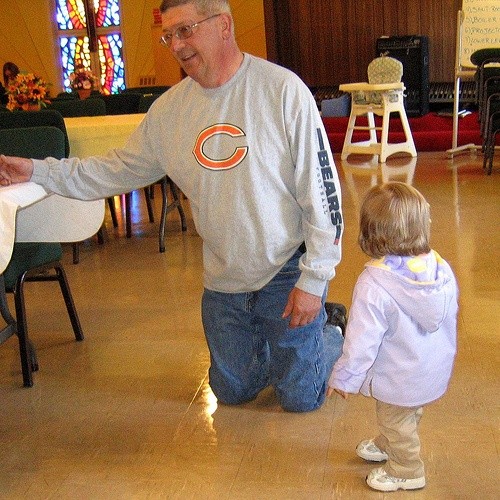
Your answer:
[376,36,430,117]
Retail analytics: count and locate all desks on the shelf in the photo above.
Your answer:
[63,113,188,253]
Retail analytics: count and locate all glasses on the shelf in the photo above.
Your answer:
[159,13,221,49]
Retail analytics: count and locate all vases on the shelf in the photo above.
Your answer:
[22,104,40,111]
[77,89,91,100]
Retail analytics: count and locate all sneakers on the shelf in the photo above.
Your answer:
[355,437,389,461]
[365,464,426,491]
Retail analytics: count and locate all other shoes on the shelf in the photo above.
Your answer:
[324,301,347,333]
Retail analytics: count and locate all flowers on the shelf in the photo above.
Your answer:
[6,73,51,112]
[70,67,100,97]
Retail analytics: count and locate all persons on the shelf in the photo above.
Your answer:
[328,181,459,491]
[0,0,350,410]
[3,61,21,86]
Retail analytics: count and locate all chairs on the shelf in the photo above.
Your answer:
[471,48,500,175]
[339,57,416,163]
[0,85,170,388]
